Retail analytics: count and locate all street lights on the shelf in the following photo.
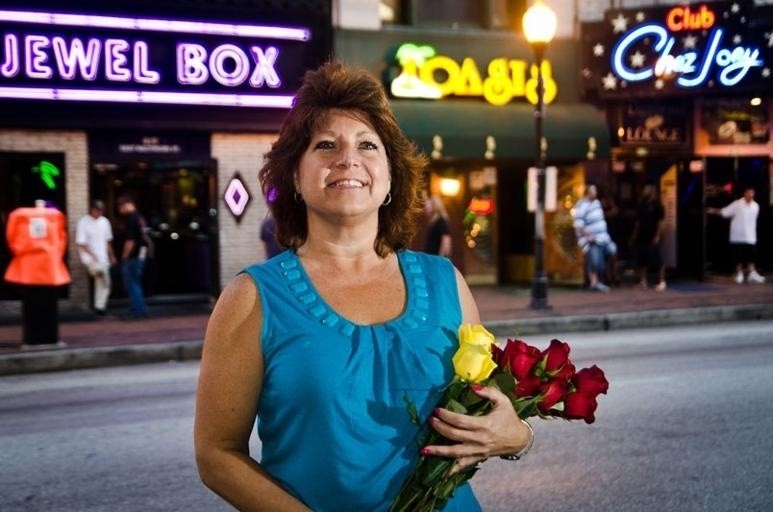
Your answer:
[516,1,566,312]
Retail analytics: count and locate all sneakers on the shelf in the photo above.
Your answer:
[733,271,745,285]
[655,281,666,293]
[746,271,767,284]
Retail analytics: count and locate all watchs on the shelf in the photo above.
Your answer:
[501,419,535,460]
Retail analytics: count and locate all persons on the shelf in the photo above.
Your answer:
[194,62,535,512]
[75,200,118,316]
[424,199,451,257]
[116,196,153,322]
[628,184,667,292]
[570,183,618,293]
[260,214,290,261]
[706,188,766,284]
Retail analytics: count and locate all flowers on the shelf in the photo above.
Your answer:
[383,318,611,512]
